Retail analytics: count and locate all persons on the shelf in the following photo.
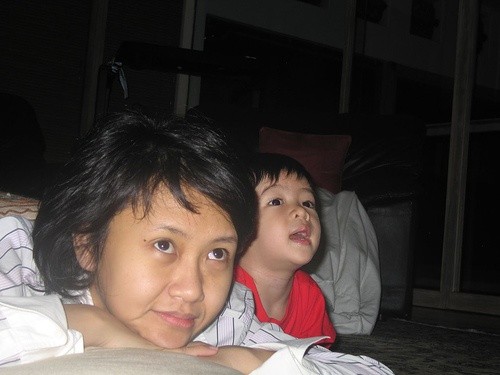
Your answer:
[232,151,337,351]
[0,104,395,375]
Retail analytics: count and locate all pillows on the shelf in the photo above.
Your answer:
[257,125,354,195]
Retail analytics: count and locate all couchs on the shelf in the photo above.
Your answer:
[188,100,426,317]
